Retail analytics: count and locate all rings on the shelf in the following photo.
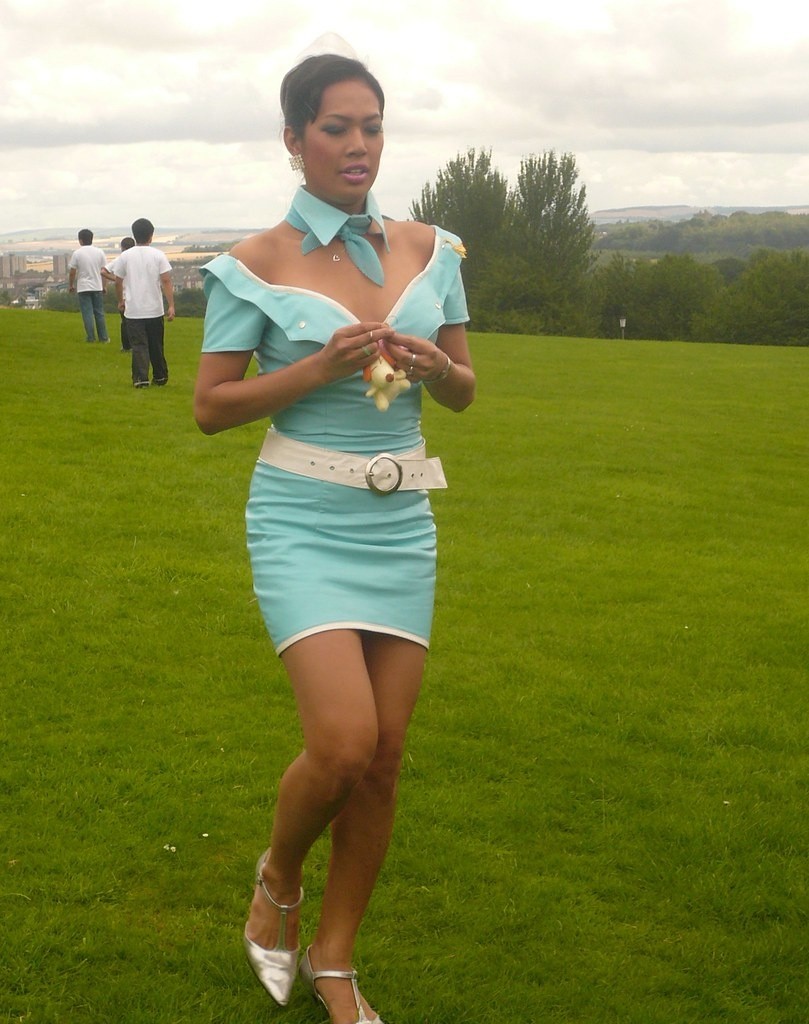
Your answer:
[410,353,416,365]
[369,330,373,343]
[362,346,372,355]
[407,367,414,375]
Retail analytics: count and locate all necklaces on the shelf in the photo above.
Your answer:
[327,245,346,262]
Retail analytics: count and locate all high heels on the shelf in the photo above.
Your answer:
[242,847,302,1007]
[300,939,384,1024]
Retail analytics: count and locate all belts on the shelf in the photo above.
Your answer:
[257,427,450,496]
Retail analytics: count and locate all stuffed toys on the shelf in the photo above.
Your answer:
[363,352,410,413]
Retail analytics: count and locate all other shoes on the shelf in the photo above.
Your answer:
[155,379,166,386]
[120,347,132,353]
[86,339,95,343]
[137,382,148,389]
[99,338,110,345]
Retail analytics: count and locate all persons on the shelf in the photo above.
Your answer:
[115,218,176,388]
[101,238,135,352]
[68,229,111,343]
[194,55,475,1024]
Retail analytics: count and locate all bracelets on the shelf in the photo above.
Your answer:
[422,357,450,385]
